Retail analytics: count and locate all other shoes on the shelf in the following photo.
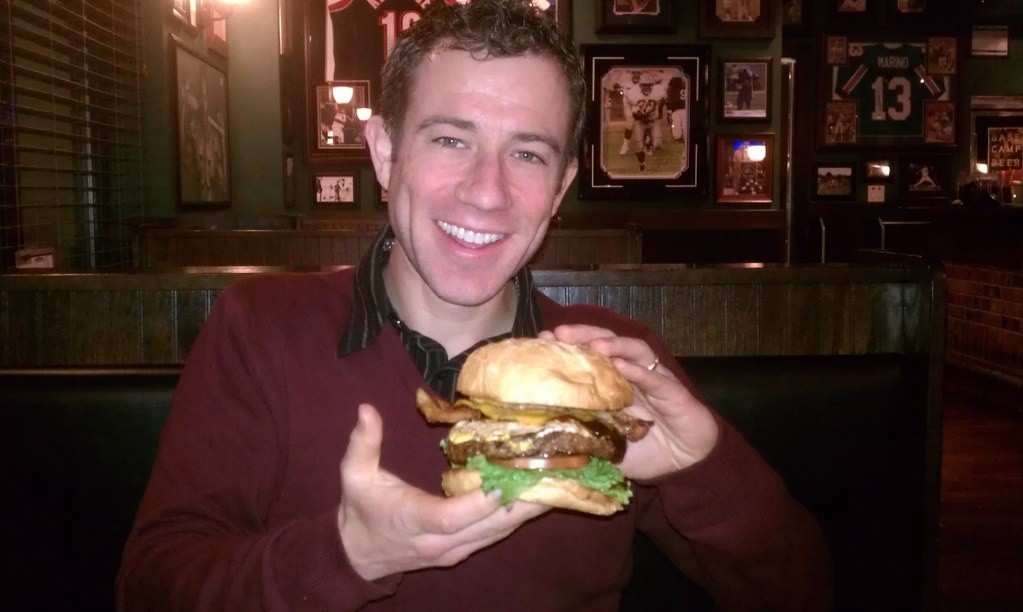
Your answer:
[644,143,653,155]
[639,162,646,172]
[619,145,629,154]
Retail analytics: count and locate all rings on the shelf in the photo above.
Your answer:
[648,357,659,370]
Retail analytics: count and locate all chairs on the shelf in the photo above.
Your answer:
[818,207,850,263]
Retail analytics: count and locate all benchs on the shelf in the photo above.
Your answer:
[0,351,925,612]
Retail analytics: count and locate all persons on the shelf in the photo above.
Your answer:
[789,1,800,23]
[732,145,745,195]
[938,41,954,73]
[931,112,952,140]
[830,41,844,61]
[316,176,345,202]
[732,65,757,110]
[613,66,686,170]
[620,0,656,12]
[331,103,356,144]
[117,0,830,612]
[722,0,751,20]
[827,107,853,142]
[914,166,936,187]
[817,173,847,194]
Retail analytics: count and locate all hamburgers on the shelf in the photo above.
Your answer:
[416,336,655,514]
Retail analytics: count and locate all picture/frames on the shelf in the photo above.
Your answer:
[310,78,371,157]
[593,0,679,35]
[280,70,296,143]
[716,55,774,125]
[308,166,361,211]
[712,132,775,207]
[167,33,234,214]
[697,0,777,41]
[824,99,860,146]
[278,0,295,63]
[578,42,710,203]
[282,151,299,210]
[921,99,957,147]
[810,161,857,201]
[898,154,954,201]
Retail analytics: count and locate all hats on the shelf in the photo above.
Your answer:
[639,72,652,85]
